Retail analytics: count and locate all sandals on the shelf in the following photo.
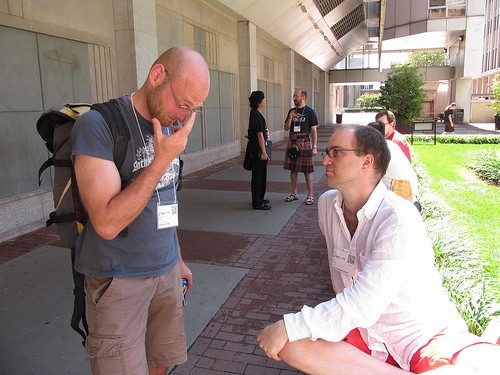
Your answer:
[306,197,315,205]
[285,193,299,202]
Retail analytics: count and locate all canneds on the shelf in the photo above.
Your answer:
[181,279,187,300]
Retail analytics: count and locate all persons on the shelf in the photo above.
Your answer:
[247,91,274,208]
[442,103,456,134]
[256,124,500,375]
[283,89,319,205]
[367,122,418,206]
[67,46,210,375]
[375,110,412,161]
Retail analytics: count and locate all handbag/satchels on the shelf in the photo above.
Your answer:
[242,141,264,170]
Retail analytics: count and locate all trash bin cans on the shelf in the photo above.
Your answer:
[494,115,500,130]
[336,113,342,123]
[454,109,464,122]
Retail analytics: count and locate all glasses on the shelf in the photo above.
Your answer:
[321,147,367,160]
[165,68,204,114]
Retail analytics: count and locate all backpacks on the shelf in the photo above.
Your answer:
[34,100,129,347]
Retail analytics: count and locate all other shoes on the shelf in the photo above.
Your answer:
[255,204,272,210]
[264,199,270,204]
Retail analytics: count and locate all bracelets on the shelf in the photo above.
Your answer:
[312,146,316,148]
